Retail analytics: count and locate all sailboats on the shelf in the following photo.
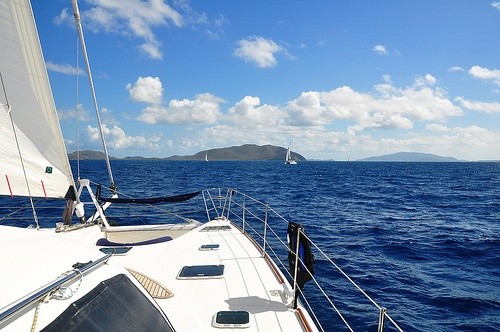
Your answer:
[0,0,406,331]
[284,146,297,165]
[205,153,209,162]
